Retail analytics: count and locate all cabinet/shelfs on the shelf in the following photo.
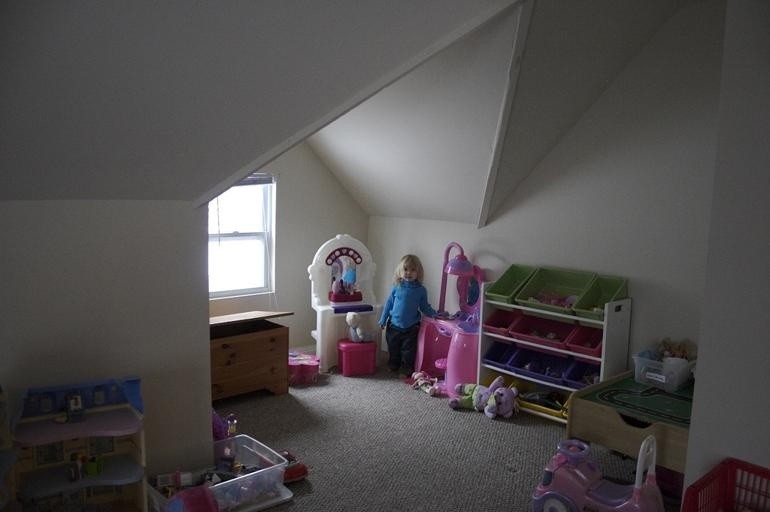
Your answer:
[8,376,148,511]
[478,281,631,424]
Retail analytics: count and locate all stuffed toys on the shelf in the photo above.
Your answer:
[409,368,439,394]
[447,376,520,421]
[343,308,367,342]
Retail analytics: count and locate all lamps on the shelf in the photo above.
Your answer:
[438,242,473,313]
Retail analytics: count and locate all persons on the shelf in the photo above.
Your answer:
[378,251,443,377]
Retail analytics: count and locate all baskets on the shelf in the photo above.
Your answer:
[683,457,770,512]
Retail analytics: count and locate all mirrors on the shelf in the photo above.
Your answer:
[456,265,484,315]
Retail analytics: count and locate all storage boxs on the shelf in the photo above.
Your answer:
[146,434,289,512]
[337,340,377,377]
[630,347,696,393]
[483,263,628,419]
[209,310,294,400]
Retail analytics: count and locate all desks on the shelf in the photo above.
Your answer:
[566,371,694,474]
